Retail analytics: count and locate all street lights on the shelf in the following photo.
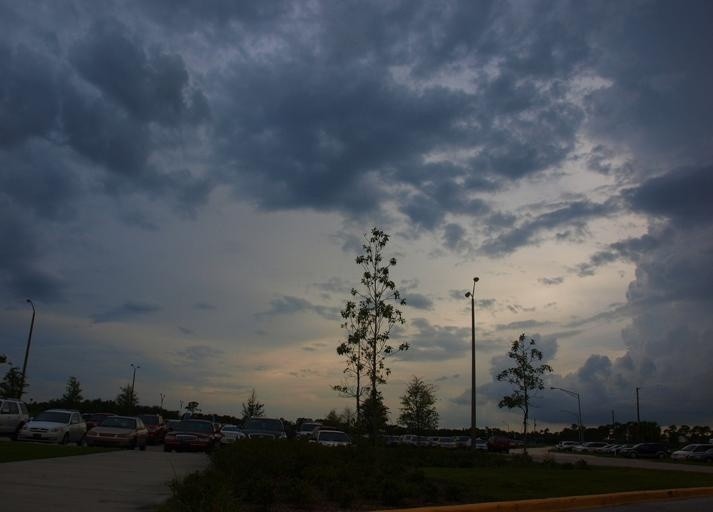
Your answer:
[130,364,140,393]
[160,392,165,408]
[17,299,35,399]
[550,386,582,439]
[464,277,479,432]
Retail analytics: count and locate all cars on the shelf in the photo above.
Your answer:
[555,440,713,463]
